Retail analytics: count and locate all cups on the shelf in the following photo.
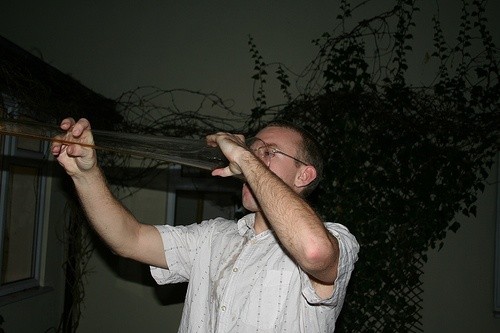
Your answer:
[0,120,269,181]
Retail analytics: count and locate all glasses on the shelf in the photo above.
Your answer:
[251,144,312,169]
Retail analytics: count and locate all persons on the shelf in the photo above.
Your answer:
[50,118,361,333]
